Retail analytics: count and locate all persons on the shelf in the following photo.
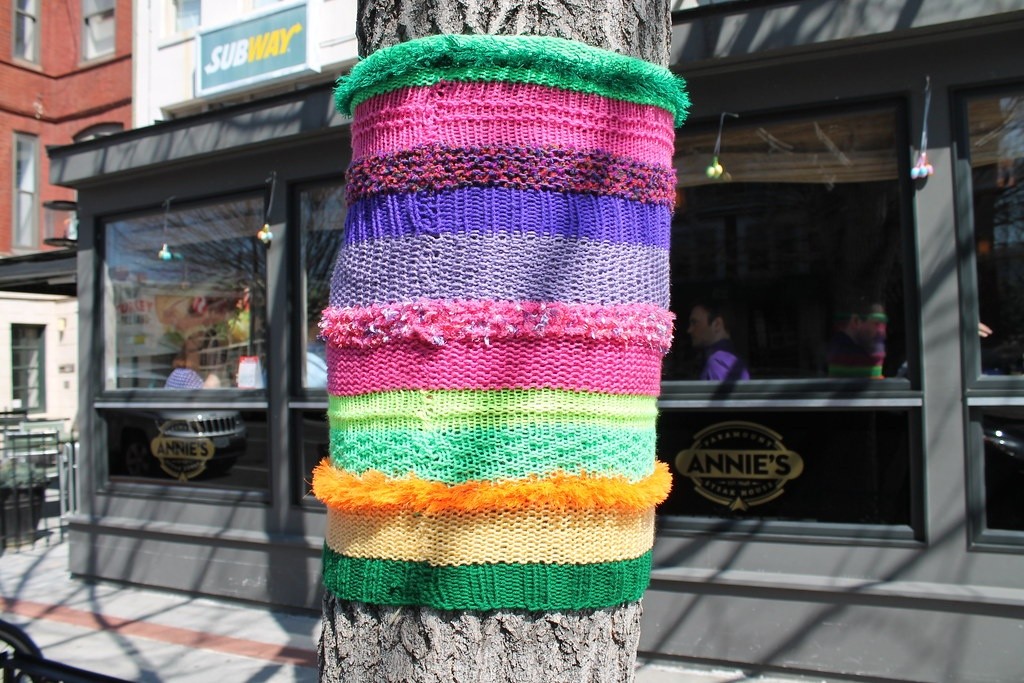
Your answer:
[687,299,748,379]
[819,297,907,376]
[164,333,220,388]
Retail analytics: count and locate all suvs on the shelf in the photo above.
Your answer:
[106,409,249,480]
